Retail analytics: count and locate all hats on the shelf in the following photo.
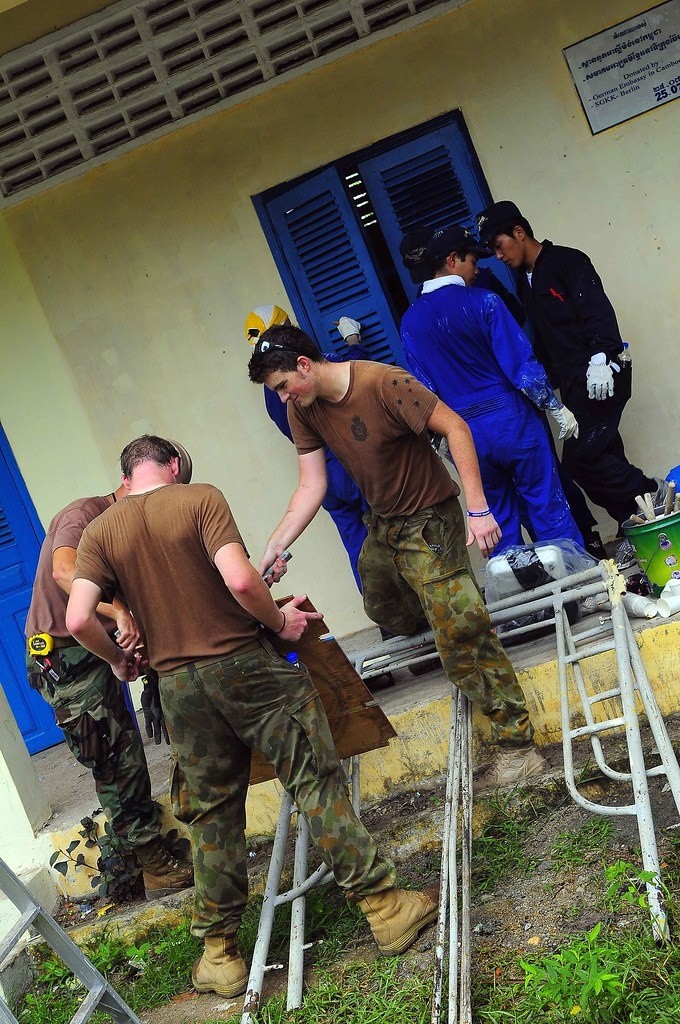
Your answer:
[398,228,438,284]
[427,227,495,263]
[475,201,522,248]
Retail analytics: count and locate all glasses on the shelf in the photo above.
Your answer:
[252,340,304,355]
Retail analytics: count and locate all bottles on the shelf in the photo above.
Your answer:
[617,341,632,369]
[286,652,303,669]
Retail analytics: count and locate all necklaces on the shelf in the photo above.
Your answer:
[111,492,116,502]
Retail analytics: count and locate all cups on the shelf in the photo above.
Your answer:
[596,590,657,617]
[657,596,680,618]
[660,578,680,598]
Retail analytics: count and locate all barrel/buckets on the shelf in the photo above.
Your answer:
[621,502,680,598]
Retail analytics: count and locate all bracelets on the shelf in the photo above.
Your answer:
[275,611,286,632]
[467,505,492,517]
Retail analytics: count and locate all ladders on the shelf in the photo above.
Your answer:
[0,858,141,1024]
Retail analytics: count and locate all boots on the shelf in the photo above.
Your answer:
[192,928,250,999]
[357,884,451,957]
[133,834,195,902]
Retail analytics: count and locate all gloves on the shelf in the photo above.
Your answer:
[140,670,170,745]
[585,353,621,401]
[550,404,579,441]
[337,317,362,342]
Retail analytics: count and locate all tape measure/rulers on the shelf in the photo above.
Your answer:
[28,631,54,656]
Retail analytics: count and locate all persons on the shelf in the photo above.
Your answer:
[248,326,550,793]
[66,434,450,999]
[245,304,399,640]
[398,228,586,577]
[25,440,196,901]
[475,201,673,570]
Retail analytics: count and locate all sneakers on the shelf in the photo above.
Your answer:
[472,743,551,793]
[408,649,442,676]
[613,537,637,570]
[637,477,668,515]
